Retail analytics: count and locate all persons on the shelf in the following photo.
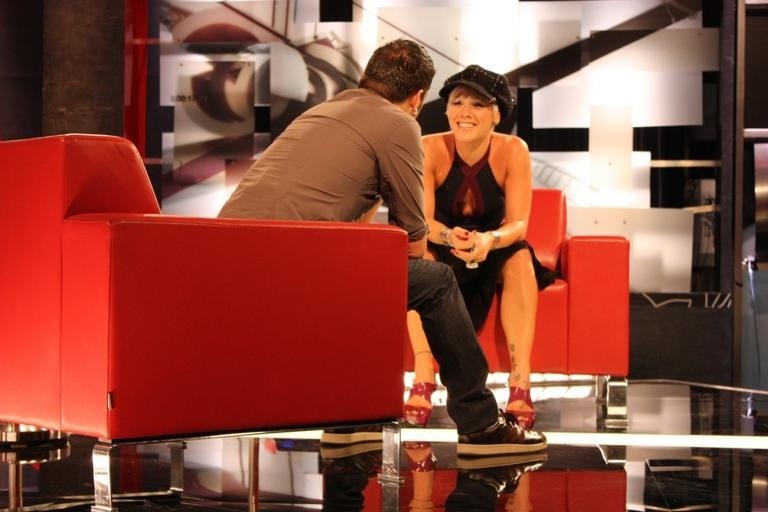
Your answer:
[216,39,547,456]
[405,65,555,430]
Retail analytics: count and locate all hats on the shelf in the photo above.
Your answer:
[439,65,513,122]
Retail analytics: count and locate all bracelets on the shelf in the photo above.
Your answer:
[440,226,452,247]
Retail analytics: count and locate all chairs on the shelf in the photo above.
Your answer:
[363,443,628,512]
[0,133,409,511]
[403,189,634,429]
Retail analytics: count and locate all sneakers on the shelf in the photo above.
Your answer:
[320,439,382,475]
[457,416,548,455]
[457,450,548,497]
[320,424,384,444]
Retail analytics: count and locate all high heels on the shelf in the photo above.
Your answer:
[403,383,437,426]
[403,441,437,472]
[507,385,536,430]
